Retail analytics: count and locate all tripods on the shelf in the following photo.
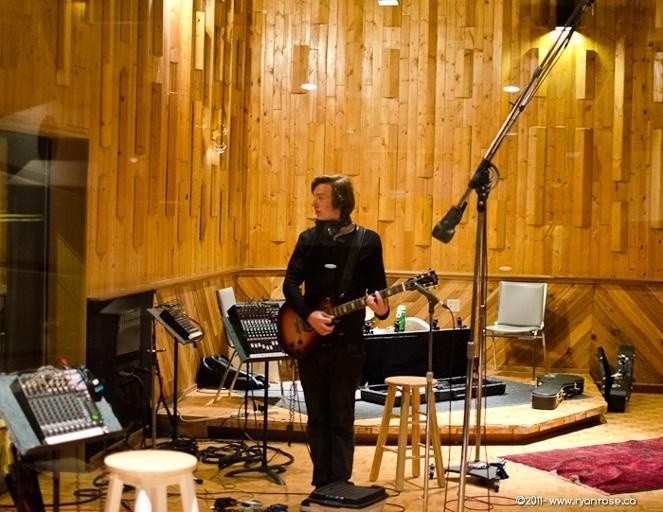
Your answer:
[224,361,286,487]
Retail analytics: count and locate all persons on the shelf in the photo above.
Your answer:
[279,173,390,491]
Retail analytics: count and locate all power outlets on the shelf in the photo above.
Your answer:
[447,300,460,312]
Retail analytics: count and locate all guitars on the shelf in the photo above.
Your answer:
[277,267,438,353]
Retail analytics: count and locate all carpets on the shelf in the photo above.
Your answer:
[499,438,663,494]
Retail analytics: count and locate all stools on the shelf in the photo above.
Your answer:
[369,376,446,487]
[103,449,198,511]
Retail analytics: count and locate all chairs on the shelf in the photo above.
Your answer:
[485,281,548,380]
[216,287,279,381]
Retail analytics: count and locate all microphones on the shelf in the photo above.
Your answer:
[409,278,444,306]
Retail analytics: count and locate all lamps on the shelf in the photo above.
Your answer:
[556,0,577,32]
